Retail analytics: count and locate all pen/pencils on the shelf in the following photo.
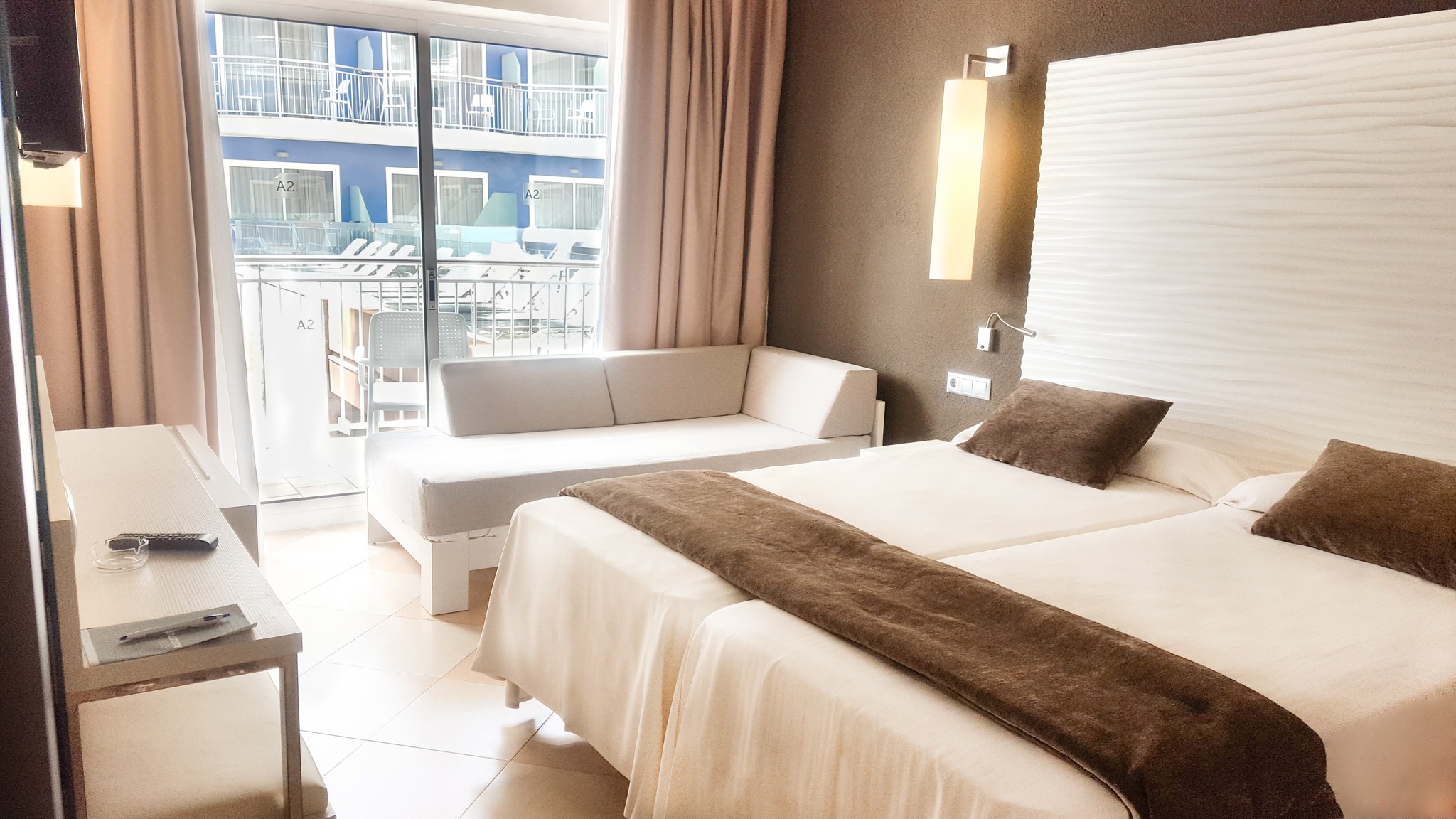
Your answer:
[120,613,232,641]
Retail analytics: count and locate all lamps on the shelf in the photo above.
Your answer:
[929,45,1010,280]
[275,152,580,174]
[976,311,1037,351]
[17,130,80,208]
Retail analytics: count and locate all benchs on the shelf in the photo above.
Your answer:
[73,670,339,819]
[363,345,885,616]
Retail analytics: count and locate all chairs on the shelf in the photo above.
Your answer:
[355,311,469,436]
[230,220,601,341]
[379,79,494,130]
[524,97,594,136]
[318,79,354,123]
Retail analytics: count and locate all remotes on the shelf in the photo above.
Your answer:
[108,532,218,551]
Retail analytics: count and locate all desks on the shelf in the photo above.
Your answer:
[36,356,303,819]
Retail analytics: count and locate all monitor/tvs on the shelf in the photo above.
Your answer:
[0,0,86,169]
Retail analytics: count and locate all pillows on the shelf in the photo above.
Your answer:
[955,378,1173,490]
[1251,438,1456,589]
[1210,472,1306,513]
[1117,434,1250,502]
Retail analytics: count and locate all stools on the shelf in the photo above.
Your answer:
[237,95,262,115]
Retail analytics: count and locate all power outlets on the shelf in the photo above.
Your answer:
[947,372,992,400]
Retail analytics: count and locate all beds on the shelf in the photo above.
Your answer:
[651,512,1456,819]
[471,441,1215,819]
